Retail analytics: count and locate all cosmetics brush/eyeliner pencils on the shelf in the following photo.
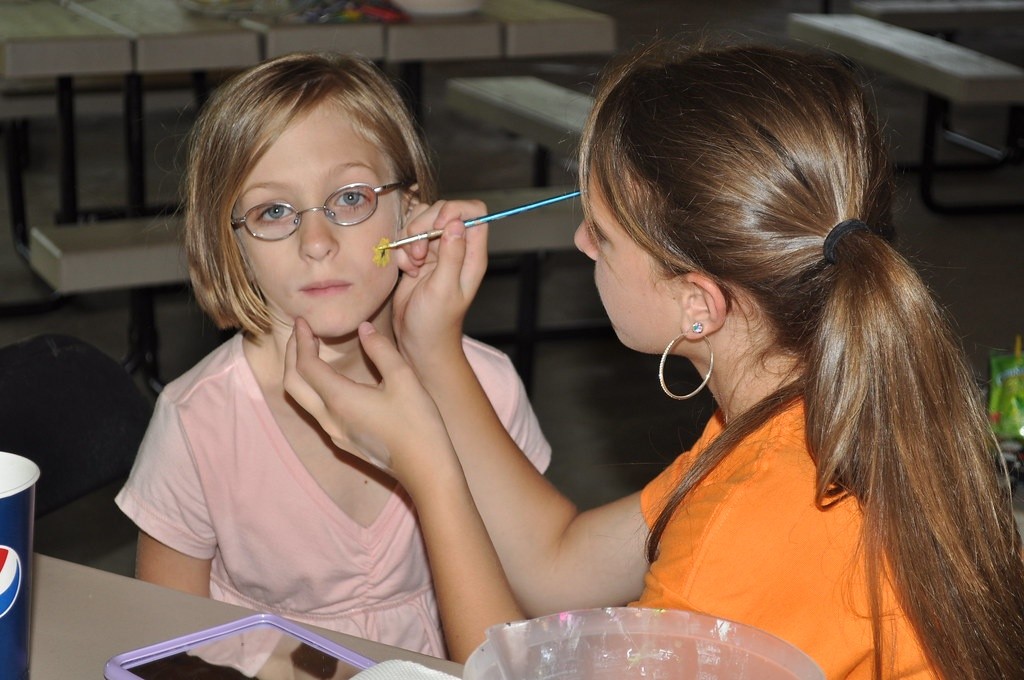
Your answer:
[379,189,585,255]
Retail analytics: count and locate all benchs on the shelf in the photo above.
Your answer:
[0,0,1024,403]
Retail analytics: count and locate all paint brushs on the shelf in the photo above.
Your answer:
[373,190,589,253]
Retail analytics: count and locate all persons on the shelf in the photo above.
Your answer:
[186,620,362,680]
[113,53,551,667]
[283,42,1024,680]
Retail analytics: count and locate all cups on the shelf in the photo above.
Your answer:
[0,450,41,680]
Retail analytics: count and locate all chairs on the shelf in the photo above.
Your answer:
[0,332,162,564]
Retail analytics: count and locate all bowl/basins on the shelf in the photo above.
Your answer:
[462,607,825,680]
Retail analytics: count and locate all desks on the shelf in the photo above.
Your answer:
[24,552,491,680]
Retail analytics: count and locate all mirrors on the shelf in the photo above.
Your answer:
[103,613,379,680]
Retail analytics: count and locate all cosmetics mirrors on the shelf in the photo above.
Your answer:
[105,613,376,680]
